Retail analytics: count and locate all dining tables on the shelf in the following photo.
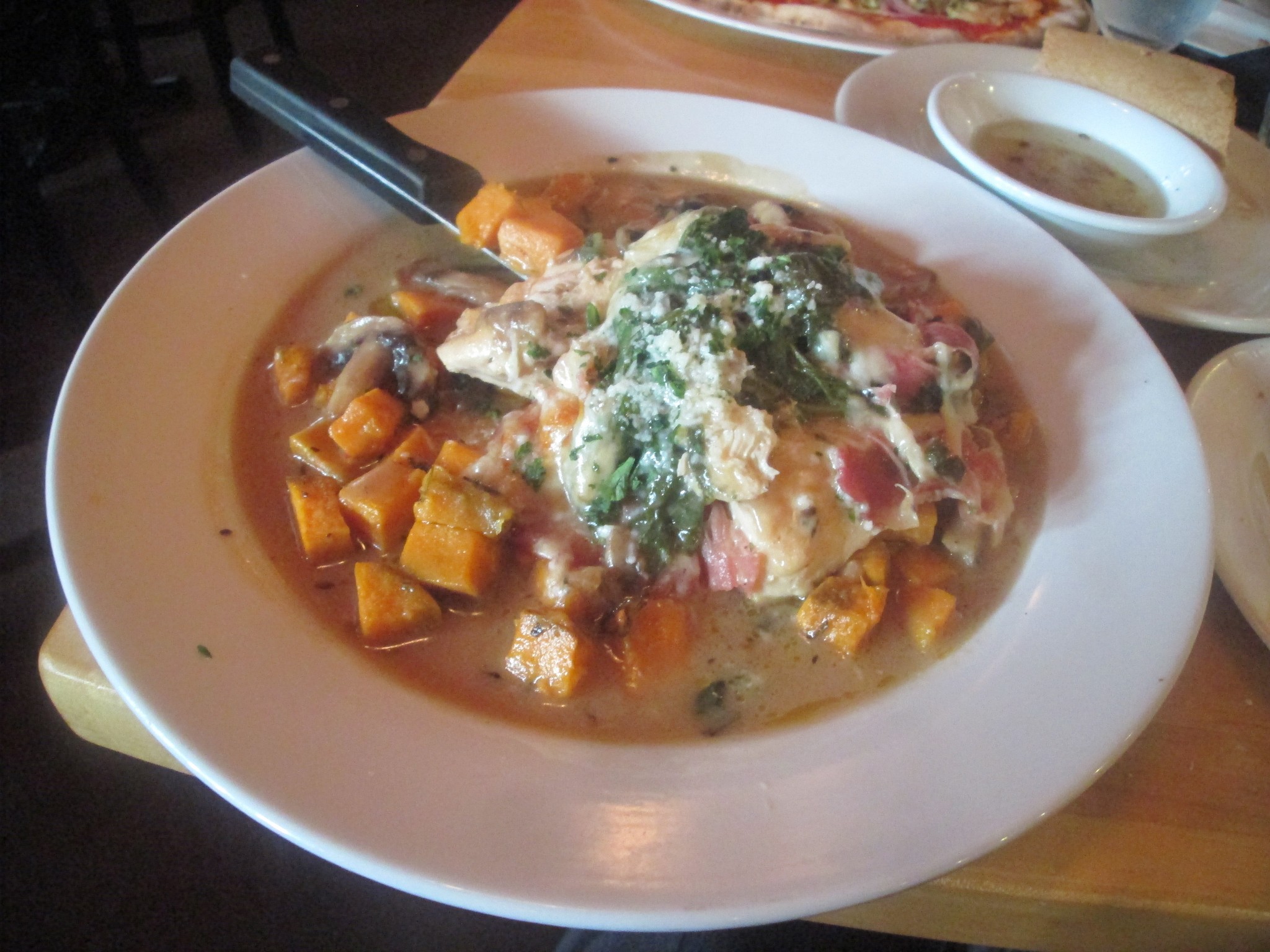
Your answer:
[38,1,1270,952]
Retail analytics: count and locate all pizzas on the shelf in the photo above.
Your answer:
[716,0,1093,49]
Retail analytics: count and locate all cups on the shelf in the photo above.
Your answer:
[1091,0,1219,54]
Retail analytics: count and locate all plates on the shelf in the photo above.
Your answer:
[832,42,1270,335]
[44,87,1217,933]
[648,0,1098,56]
[1184,337,1270,645]
[1179,0,1270,57]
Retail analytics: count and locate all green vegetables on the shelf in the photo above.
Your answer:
[469,196,1001,741]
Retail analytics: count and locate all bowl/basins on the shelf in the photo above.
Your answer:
[925,72,1228,261]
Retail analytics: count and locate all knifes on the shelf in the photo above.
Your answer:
[225,43,533,281]
